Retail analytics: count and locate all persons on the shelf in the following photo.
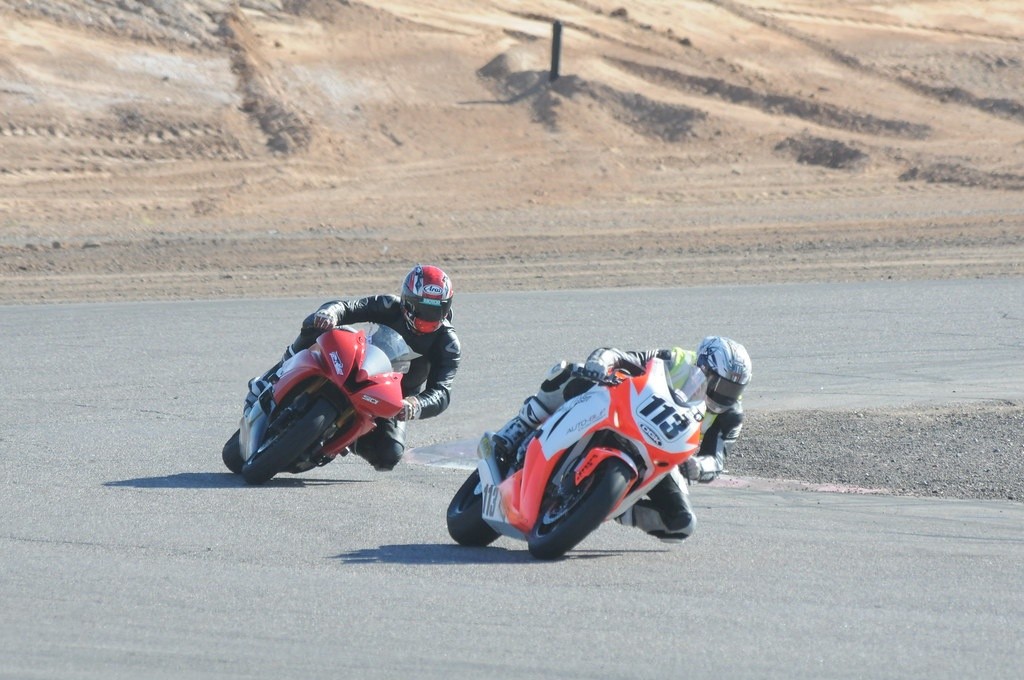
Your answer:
[491,334,752,544]
[244,265,461,472]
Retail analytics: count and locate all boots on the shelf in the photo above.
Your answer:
[492,395,553,456]
[348,439,358,455]
[613,497,642,527]
[248,344,296,398]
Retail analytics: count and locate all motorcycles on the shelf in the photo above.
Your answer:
[222,324,409,485]
[447,366,708,560]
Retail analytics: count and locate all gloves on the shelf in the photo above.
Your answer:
[583,359,607,384]
[678,456,701,480]
[314,310,338,330]
[394,396,422,422]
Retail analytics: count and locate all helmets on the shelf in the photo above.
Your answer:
[695,336,751,414]
[400,265,454,336]
